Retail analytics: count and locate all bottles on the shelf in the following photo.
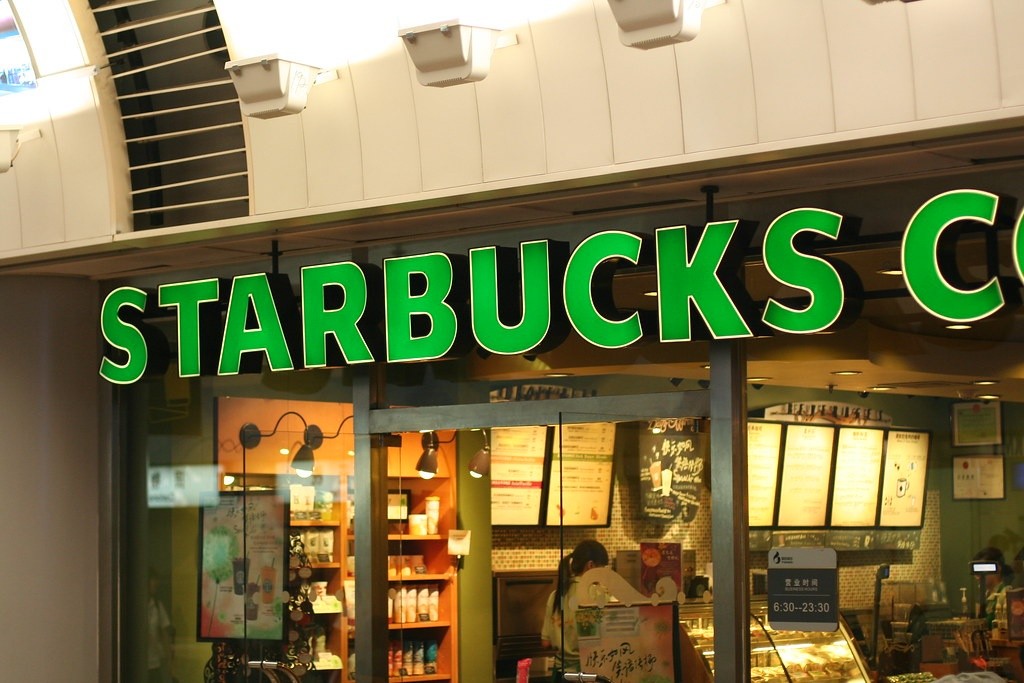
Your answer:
[388,631,438,677]
[349,640,355,680]
[388,582,439,624]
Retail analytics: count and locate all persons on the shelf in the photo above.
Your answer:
[541,540,609,683]
[971,546,1015,621]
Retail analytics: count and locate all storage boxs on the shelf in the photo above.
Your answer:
[920,657,961,676]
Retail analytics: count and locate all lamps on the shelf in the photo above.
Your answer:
[240,411,314,478]
[0,127,43,172]
[224,52,339,120]
[608,0,702,49]
[415,431,438,480]
[438,428,490,478]
[398,18,518,87]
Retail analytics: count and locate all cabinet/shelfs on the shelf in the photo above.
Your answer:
[222,473,459,683]
[677,600,876,683]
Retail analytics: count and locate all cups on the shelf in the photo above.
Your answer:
[896,477,910,498]
[241,582,260,621]
[424,496,440,535]
[408,514,427,535]
[231,558,251,595]
[661,469,673,497]
[311,581,328,597]
[649,461,661,488]
[348,555,355,577]
[259,566,278,604]
[388,555,425,577]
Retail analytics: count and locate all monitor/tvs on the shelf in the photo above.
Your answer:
[906,602,948,633]
